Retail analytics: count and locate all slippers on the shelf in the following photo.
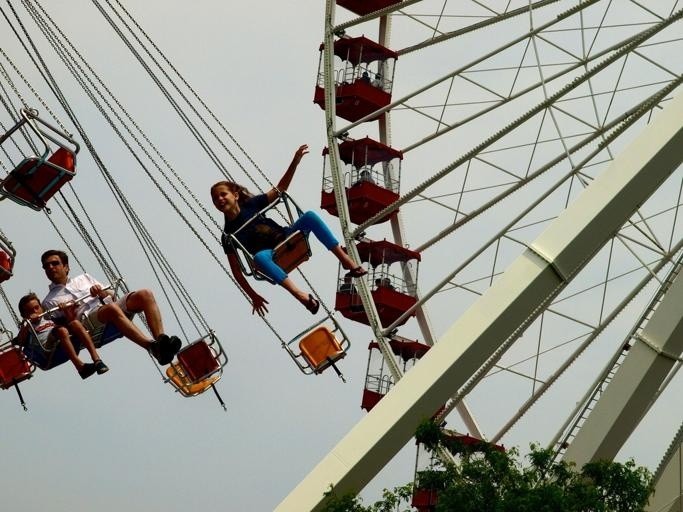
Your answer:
[307,293,320,315]
[343,266,368,278]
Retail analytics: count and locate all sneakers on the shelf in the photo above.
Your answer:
[148,334,182,365]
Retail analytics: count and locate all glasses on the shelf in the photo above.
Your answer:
[42,261,60,268]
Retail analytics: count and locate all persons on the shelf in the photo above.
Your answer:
[358,170,376,184]
[360,72,384,90]
[18,250,182,380]
[340,275,394,294]
[211,144,367,315]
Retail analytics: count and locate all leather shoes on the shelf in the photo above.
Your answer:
[79,360,107,380]
